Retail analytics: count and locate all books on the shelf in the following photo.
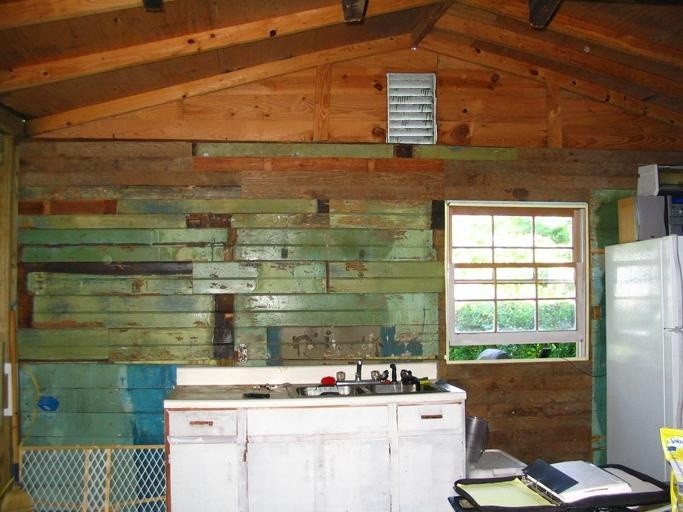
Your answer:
[458,460,663,507]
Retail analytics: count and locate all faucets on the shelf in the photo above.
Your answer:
[354,360,363,381]
[390,364,397,383]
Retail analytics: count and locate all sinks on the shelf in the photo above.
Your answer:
[285,380,368,398]
[363,381,450,398]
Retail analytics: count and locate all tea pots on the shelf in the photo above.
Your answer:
[401,370,420,393]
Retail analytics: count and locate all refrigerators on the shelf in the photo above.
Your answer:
[604,235,683,485]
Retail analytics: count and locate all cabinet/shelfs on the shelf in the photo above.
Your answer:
[397,400,467,511]
[165,409,246,512]
[248,404,397,512]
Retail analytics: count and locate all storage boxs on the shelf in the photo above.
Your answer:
[467,448,528,479]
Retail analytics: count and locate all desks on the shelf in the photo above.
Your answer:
[448,482,683,512]
